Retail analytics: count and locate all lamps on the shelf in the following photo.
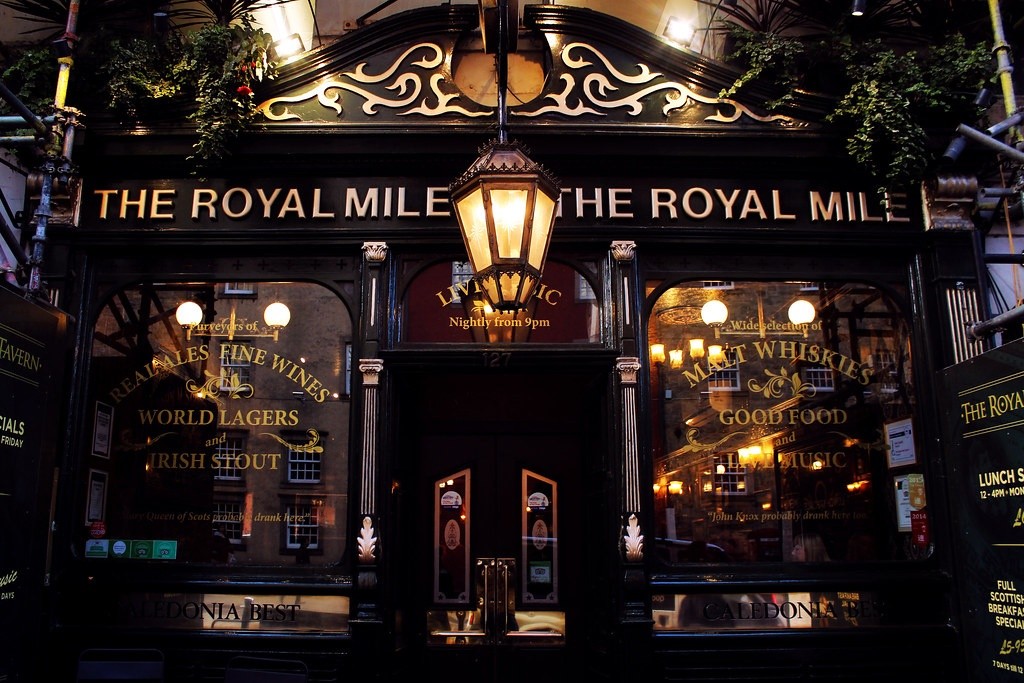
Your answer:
[702,295,817,339]
[449,1,563,316]
[647,280,731,372]
[175,301,292,343]
